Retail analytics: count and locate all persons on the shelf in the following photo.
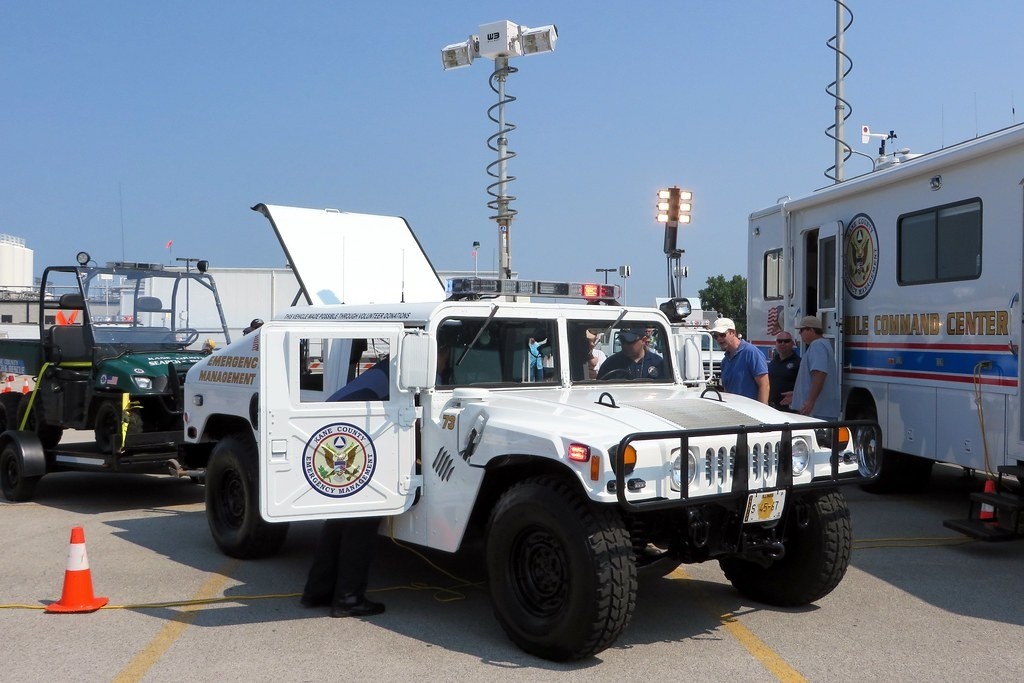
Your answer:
[586,327,664,379]
[300,357,389,616]
[527,336,547,381]
[708,316,839,423]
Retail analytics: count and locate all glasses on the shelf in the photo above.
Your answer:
[776,338,792,343]
[712,332,726,340]
[800,328,808,332]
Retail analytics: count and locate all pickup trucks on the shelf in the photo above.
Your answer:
[0,252,263,502]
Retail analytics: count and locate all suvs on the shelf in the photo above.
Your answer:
[656,298,726,386]
[183,202,883,661]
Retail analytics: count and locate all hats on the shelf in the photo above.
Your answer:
[615,328,646,341]
[795,316,822,328]
[709,318,736,333]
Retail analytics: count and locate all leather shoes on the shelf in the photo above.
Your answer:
[301,595,332,606]
[331,599,386,617]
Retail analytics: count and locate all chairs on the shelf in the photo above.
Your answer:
[50,325,101,361]
[124,327,172,351]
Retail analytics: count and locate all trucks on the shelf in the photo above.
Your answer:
[747,117,1024,499]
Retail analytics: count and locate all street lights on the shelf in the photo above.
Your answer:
[441,20,558,279]
[595,268,617,285]
[176,258,199,329]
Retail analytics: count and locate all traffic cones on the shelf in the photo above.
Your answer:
[979,481,1000,524]
[46,526,109,613]
